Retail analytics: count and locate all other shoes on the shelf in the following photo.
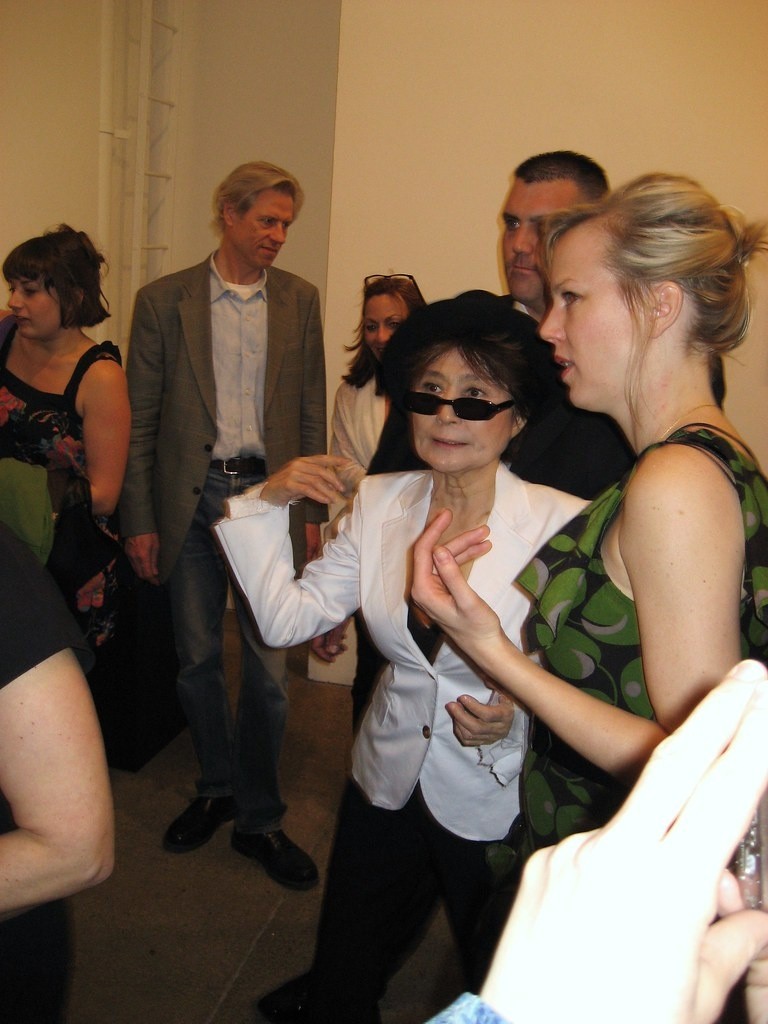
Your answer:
[257,969,380,1024]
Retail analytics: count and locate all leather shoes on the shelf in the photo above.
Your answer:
[163,788,239,852]
[231,821,319,890]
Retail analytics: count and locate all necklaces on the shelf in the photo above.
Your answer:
[660,403,719,438]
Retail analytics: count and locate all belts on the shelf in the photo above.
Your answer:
[212,456,268,477]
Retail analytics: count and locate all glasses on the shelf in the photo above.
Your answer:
[365,272,424,303]
[400,390,519,420]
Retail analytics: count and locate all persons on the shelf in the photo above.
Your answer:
[327,152,639,739]
[405,174,768,861]
[429,658,768,1024]
[0,521,117,1024]
[0,221,135,695]
[208,290,598,1024]
[126,160,327,894]
